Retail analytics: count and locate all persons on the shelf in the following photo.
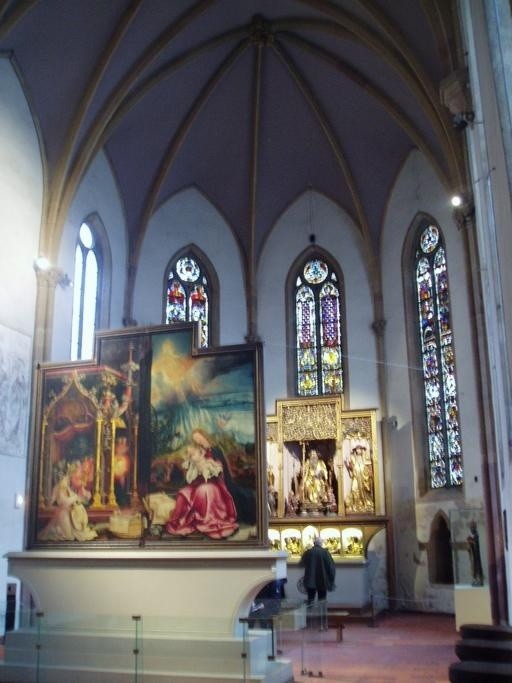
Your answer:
[301,449,328,503]
[345,445,370,506]
[298,537,335,604]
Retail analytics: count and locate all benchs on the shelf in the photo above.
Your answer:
[305,611,351,643]
